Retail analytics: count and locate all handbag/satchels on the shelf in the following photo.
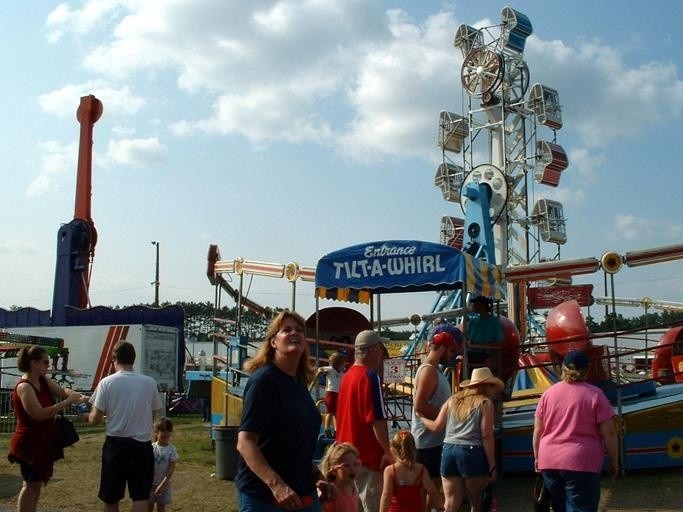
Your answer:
[531,473,550,512]
[55,418,79,448]
[479,480,497,512]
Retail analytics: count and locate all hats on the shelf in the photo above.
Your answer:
[459,367,505,393]
[563,351,588,371]
[432,332,463,352]
[470,295,490,305]
[354,330,390,351]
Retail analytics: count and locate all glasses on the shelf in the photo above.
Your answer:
[328,460,362,472]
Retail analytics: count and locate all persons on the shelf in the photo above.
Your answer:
[532,350,620,511]
[80,340,163,512]
[151,416,179,512]
[411,296,507,512]
[235,312,321,511]
[336,331,394,512]
[201,398,211,423]
[318,352,347,439]
[377,430,445,511]
[8,346,90,512]
[313,441,362,512]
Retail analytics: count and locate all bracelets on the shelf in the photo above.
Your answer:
[53,403,59,415]
